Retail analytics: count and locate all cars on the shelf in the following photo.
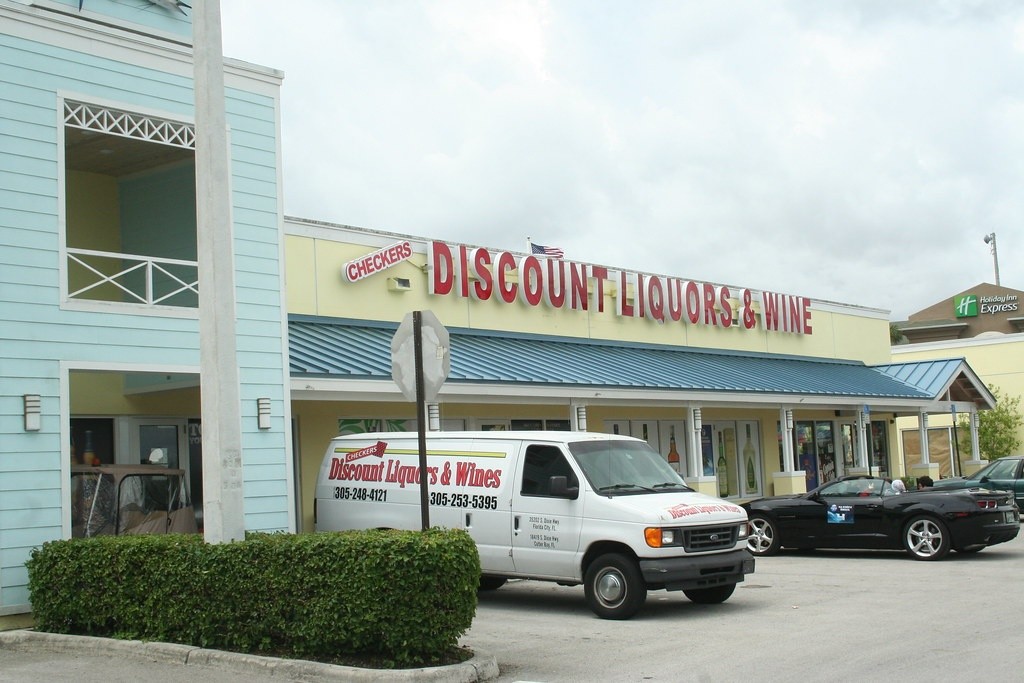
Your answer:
[907,455,1024,514]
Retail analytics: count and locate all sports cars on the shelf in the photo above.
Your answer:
[739,475,1019,562]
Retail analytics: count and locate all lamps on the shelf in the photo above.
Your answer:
[428,404,440,431]
[24,393,41,432]
[923,413,928,429]
[974,413,980,428]
[785,410,793,429]
[256,397,272,430]
[861,411,870,428]
[577,406,587,430]
[693,409,702,430]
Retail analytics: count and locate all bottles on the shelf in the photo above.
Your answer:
[70,426,79,465]
[83,430,95,465]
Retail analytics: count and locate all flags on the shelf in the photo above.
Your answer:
[531,243,564,259]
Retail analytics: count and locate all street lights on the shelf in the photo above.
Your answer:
[984,232,1000,285]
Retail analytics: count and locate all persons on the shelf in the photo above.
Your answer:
[858,477,871,496]
[891,479,906,495]
[918,476,933,489]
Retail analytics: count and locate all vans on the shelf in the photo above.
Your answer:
[313,432,756,621]
